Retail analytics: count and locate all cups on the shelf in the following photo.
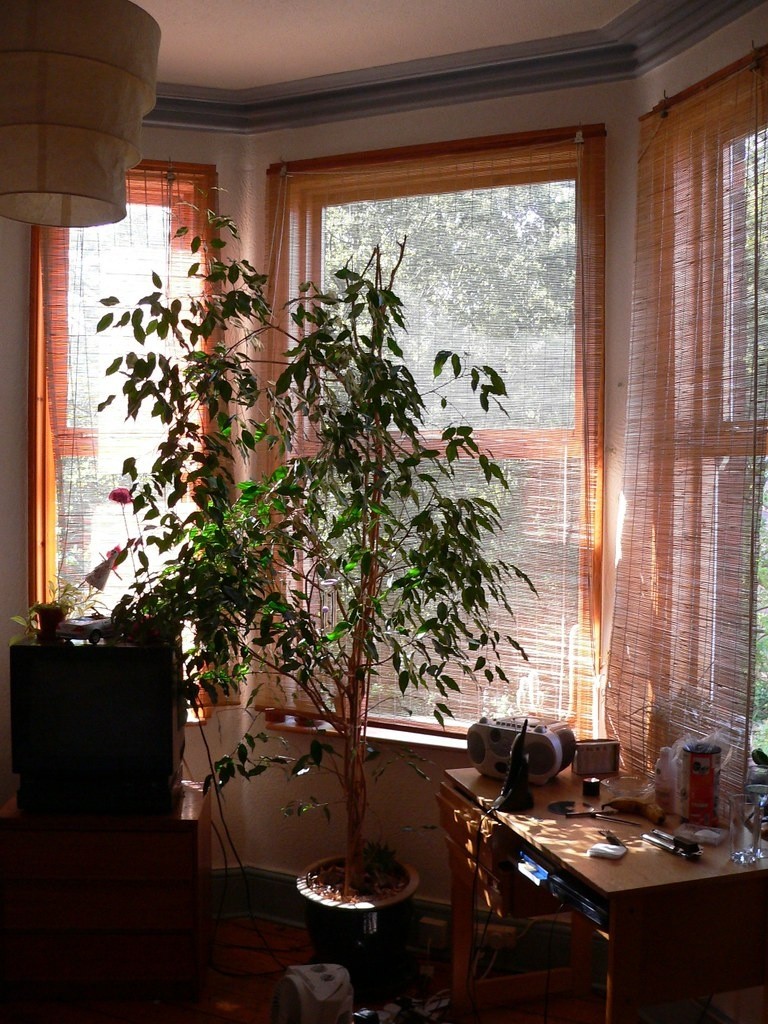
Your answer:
[729,793,760,864]
[746,785,768,827]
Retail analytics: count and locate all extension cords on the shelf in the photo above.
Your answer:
[471,924,517,955]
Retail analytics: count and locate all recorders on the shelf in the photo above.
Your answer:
[466,715,575,784]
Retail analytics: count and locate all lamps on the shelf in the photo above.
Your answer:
[0,1,167,227]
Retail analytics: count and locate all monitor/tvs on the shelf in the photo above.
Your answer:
[10,631,185,817]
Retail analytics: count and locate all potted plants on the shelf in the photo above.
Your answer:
[17,586,70,646]
[88,192,544,997]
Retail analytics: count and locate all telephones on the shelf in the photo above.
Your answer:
[491,718,534,812]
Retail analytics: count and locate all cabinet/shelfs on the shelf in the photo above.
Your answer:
[0,807,209,1022]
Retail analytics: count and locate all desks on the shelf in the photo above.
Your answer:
[19,636,189,813]
[438,758,767,1024]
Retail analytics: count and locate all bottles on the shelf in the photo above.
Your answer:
[654,746,677,814]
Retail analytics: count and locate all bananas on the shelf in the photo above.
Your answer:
[601,796,666,827]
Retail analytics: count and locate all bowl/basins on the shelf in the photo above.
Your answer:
[601,773,656,800]
